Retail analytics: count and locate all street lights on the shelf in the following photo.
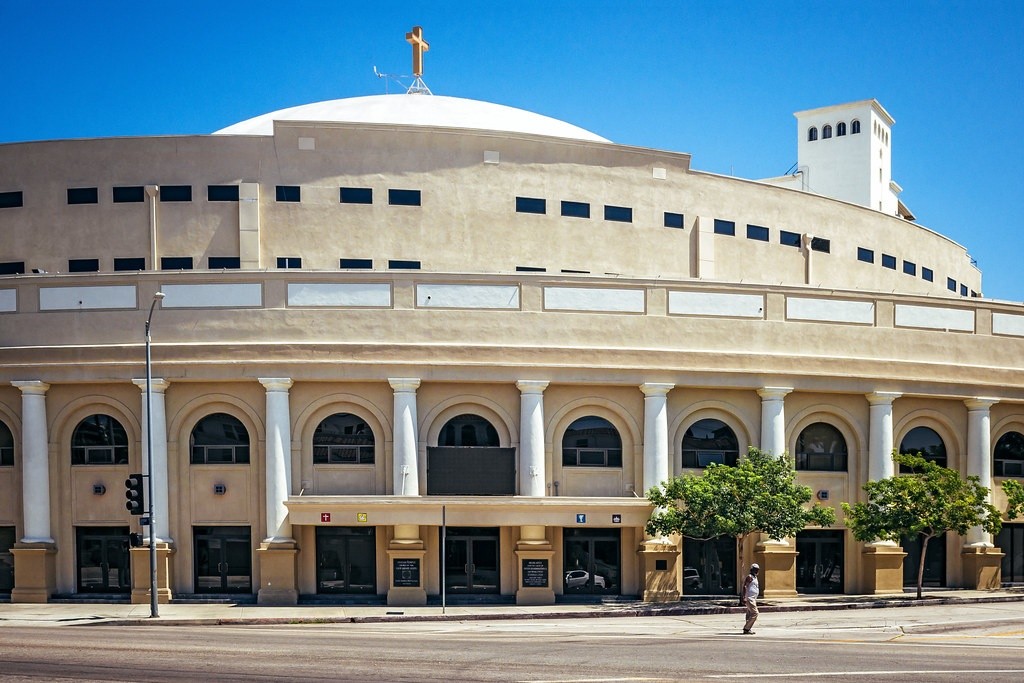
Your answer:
[145,291,167,618]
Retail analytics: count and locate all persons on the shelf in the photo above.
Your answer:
[742,563,760,635]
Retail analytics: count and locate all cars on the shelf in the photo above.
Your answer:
[564,569,606,595]
[684,568,704,592]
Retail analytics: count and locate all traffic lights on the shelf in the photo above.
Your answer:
[125,473,144,515]
